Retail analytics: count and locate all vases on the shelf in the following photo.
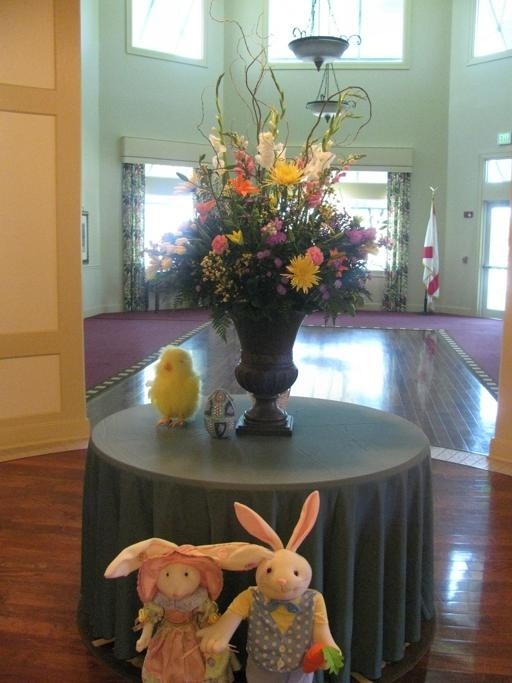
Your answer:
[228,304,313,439]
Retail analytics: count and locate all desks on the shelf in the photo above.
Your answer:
[86,395,433,681]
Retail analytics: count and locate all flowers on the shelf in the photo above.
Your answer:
[149,0,400,339]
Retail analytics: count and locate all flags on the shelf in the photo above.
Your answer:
[421,196,442,310]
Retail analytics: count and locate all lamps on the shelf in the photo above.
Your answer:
[287,0,362,124]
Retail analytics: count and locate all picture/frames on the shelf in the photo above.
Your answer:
[81,211,89,265]
